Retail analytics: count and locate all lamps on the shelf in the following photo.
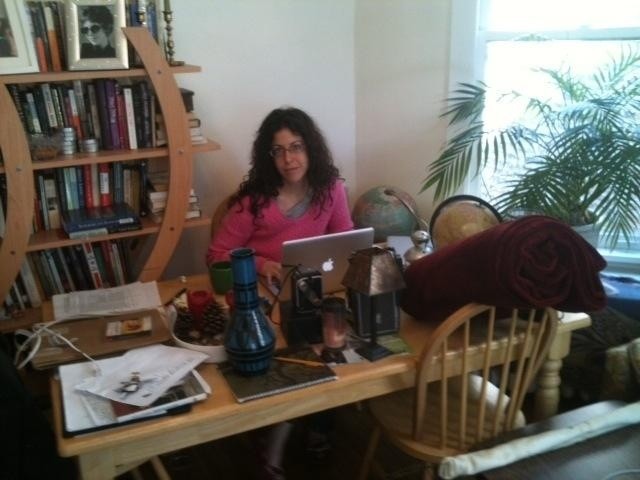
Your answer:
[339,245,408,364]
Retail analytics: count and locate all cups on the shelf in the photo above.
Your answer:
[209,258,233,295]
[320,294,349,352]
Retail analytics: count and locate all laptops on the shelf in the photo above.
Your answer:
[259,227,375,301]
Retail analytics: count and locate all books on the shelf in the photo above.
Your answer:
[0,0,171,77]
[6,80,204,159]
[2,158,203,239]
[0,239,126,317]
[111,370,208,423]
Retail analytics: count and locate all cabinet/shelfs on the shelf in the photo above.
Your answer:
[0,23,222,334]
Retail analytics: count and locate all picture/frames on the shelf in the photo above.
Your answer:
[0,0,39,77]
[67,1,128,70]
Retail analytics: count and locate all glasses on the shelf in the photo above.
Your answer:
[80,25,100,33]
[269,144,306,157]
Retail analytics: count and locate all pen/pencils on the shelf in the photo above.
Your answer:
[165,287,187,306]
[273,357,325,368]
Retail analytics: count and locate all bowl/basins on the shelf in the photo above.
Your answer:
[168,313,228,368]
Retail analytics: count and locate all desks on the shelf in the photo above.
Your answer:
[40,239,592,480]
[453,400,640,479]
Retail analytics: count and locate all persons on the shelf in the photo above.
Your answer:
[205,105,355,288]
[78,5,115,58]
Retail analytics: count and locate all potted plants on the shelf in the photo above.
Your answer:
[418,41,640,276]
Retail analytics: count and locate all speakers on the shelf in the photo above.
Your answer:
[281,304,322,346]
[348,287,399,337]
[291,267,322,314]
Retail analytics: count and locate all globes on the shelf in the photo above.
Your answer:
[351,185,419,244]
[429,195,503,252]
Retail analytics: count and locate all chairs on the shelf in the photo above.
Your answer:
[362,286,558,479]
